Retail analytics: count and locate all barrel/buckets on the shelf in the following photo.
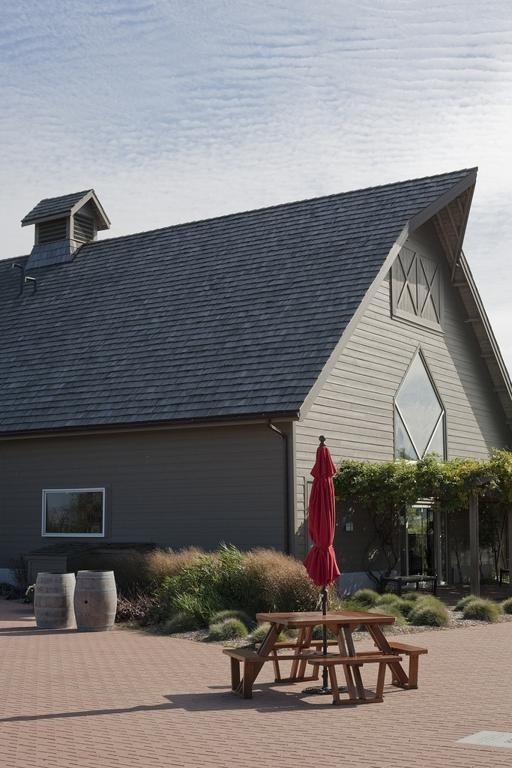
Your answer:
[34,572,75,628]
[73,570,117,630]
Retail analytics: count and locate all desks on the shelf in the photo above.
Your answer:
[235,611,410,695]
[379,573,438,597]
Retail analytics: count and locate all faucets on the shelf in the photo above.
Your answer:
[24,583,35,597]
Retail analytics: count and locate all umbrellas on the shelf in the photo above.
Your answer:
[307,433,340,684]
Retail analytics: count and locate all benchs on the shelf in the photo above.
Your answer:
[222,639,428,705]
